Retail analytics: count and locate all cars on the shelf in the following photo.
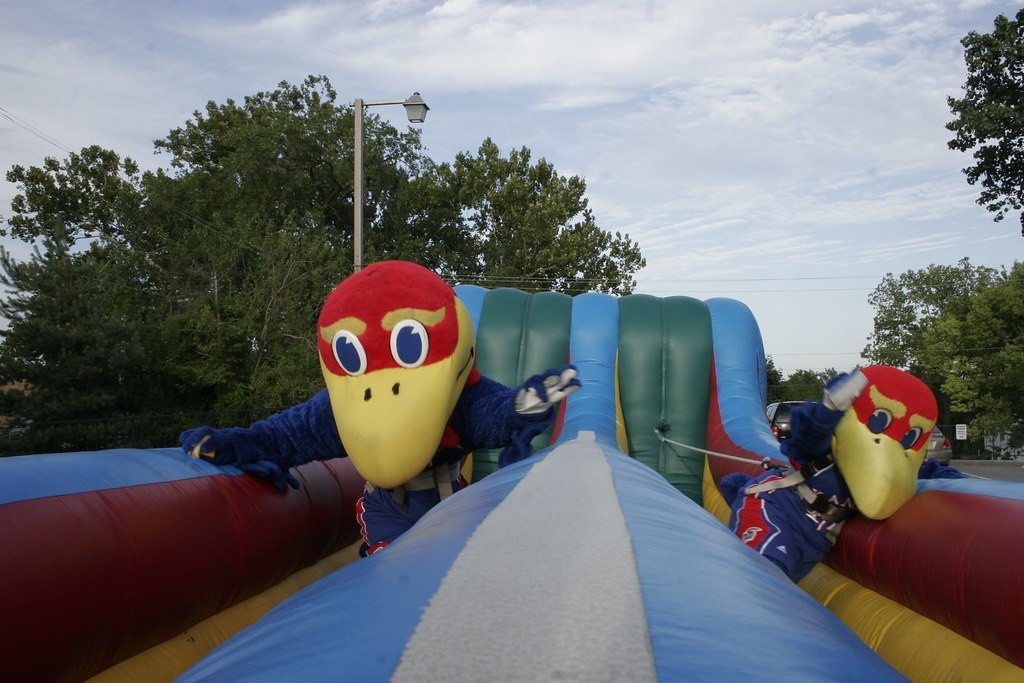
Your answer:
[924,426,952,467]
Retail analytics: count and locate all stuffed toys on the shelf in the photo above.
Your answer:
[720,365,967,585]
[179,261,582,561]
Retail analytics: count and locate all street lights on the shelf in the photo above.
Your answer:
[349,92,430,272]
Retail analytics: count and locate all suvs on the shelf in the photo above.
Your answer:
[765,401,822,442]
